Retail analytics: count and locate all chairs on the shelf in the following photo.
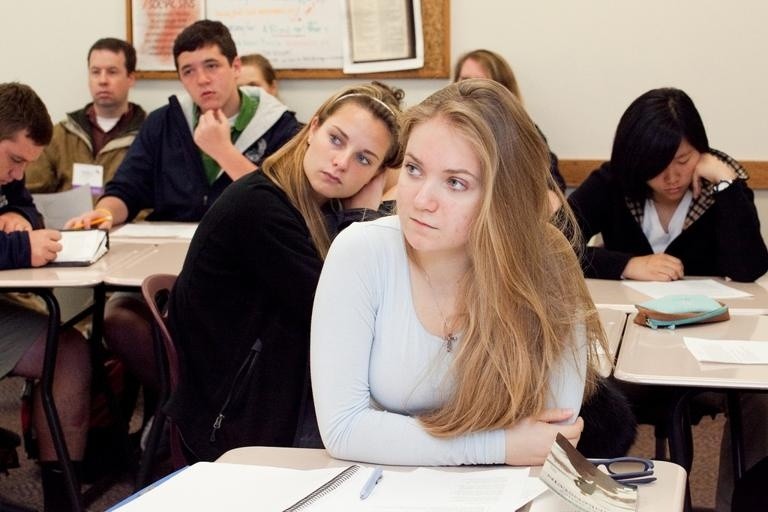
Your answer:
[138,271,185,472]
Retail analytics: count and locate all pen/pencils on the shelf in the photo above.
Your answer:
[71,216,113,231]
[359,466,383,499]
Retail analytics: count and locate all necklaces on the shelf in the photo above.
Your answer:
[406,250,460,354]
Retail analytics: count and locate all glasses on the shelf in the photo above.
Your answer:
[584,453,656,487]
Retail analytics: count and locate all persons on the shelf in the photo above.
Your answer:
[25,35,167,483]
[453,45,566,220]
[545,83,768,511]
[310,77,617,468]
[1,79,91,510]
[65,18,310,483]
[232,51,283,99]
[168,84,403,464]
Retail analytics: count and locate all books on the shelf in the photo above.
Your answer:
[111,429,640,512]
[54,223,112,267]
[111,213,204,243]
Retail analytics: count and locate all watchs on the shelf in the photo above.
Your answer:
[710,179,737,194]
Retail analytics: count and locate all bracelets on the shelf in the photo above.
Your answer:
[88,206,115,222]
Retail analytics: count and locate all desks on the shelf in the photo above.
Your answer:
[210,445,688,512]
[587,266,763,392]
[0,212,176,508]
